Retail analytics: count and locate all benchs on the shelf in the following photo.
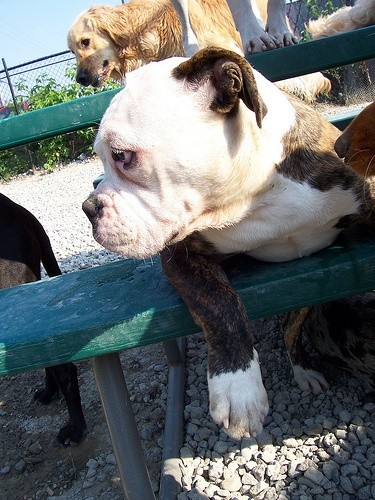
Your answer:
[0,224,375,500]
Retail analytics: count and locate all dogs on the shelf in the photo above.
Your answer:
[0,192,90,449]
[67,0,375,102]
[80,45,375,442]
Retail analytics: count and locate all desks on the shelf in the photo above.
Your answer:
[0,25,375,153]
